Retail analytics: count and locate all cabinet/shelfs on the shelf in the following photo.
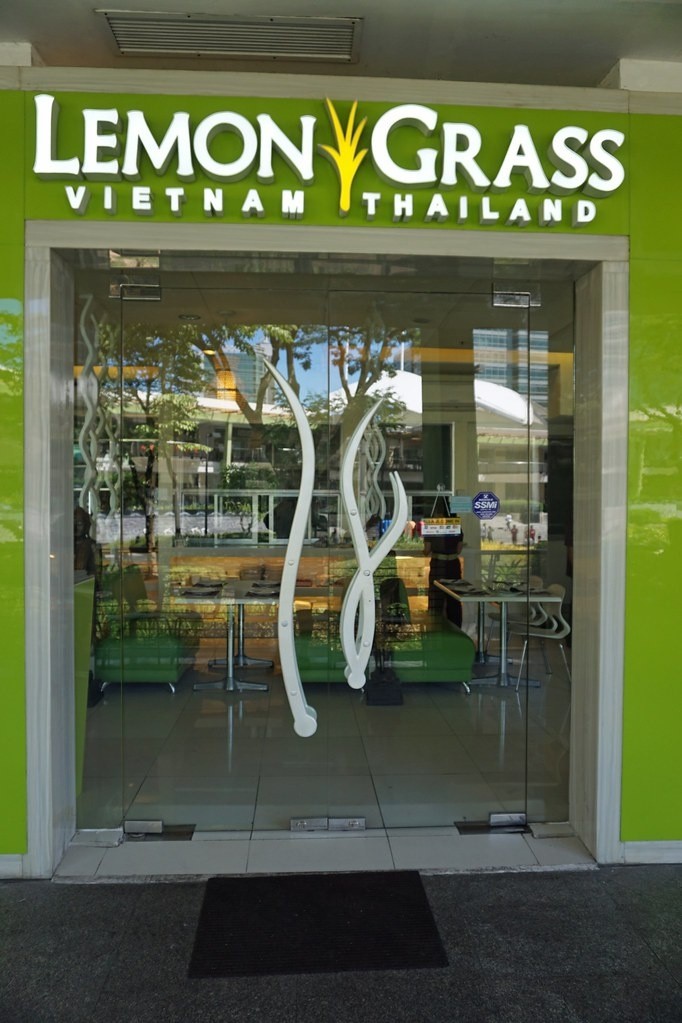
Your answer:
[101,547,429,637]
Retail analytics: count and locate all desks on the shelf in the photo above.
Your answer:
[175,580,279,692]
[433,578,562,688]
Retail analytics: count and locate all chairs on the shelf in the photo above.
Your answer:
[508,584,572,691]
[484,575,550,674]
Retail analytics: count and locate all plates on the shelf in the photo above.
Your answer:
[442,579,546,593]
[186,579,279,595]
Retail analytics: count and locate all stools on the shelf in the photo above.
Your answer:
[295,616,476,695]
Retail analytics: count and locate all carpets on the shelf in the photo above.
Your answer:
[187,870,449,978]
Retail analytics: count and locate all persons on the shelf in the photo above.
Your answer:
[264,467,320,669]
[481,518,542,545]
[74,507,104,708]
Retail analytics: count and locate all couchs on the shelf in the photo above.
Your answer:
[86,563,202,694]
